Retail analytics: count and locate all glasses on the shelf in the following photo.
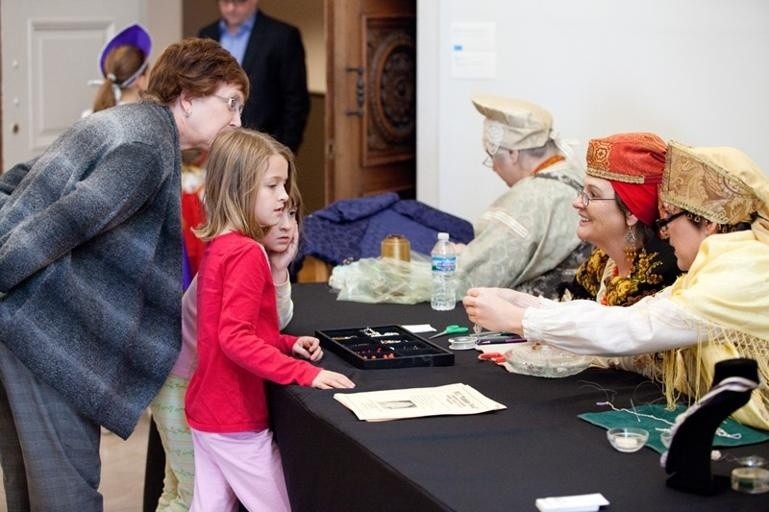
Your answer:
[210,91,245,115]
[655,210,687,233]
[483,146,497,170]
[575,187,616,206]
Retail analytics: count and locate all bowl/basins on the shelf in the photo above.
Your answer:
[606,426,650,453]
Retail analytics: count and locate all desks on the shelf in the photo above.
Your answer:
[142,280,764,511]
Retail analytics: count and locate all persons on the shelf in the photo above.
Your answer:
[147,185,299,512]
[461,139,769,432]
[555,132,688,307]
[430,97,583,302]
[198,0,311,158]
[79,25,152,120]
[0,38,250,511]
[183,128,355,511]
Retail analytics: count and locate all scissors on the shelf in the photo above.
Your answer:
[428,324,468,340]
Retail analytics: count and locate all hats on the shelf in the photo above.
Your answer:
[660,139,769,234]
[584,131,669,226]
[469,93,556,150]
[97,21,155,87]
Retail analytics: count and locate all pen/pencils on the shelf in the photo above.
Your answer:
[480,339,527,345]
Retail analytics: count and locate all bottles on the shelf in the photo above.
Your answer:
[381,233,411,297]
[430,232,456,312]
